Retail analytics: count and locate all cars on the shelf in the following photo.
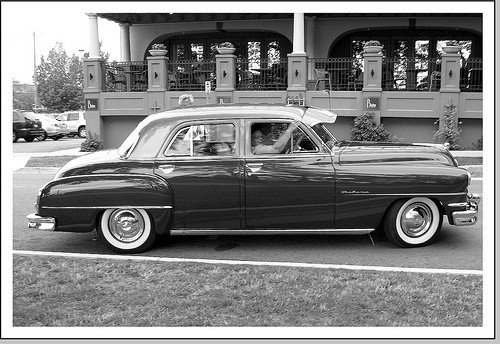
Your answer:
[26,104,481,256]
[36,110,86,140]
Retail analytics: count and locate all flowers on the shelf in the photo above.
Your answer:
[151,43,166,50]
[364,40,381,46]
[218,41,234,48]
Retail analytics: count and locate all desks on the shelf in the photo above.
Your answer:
[194,70,214,81]
[404,68,428,91]
[251,68,273,90]
[328,67,350,90]
[122,70,141,91]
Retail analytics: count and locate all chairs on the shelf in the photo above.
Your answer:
[237,64,287,91]
[383,64,407,91]
[428,71,441,91]
[312,68,333,93]
[169,69,194,91]
[108,68,148,91]
[347,68,363,90]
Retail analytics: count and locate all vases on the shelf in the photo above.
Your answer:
[362,45,385,53]
[149,49,168,57]
[216,48,236,54]
[441,45,462,54]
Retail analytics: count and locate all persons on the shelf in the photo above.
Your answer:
[251,121,297,155]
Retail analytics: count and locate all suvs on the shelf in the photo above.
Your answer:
[13,109,44,143]
